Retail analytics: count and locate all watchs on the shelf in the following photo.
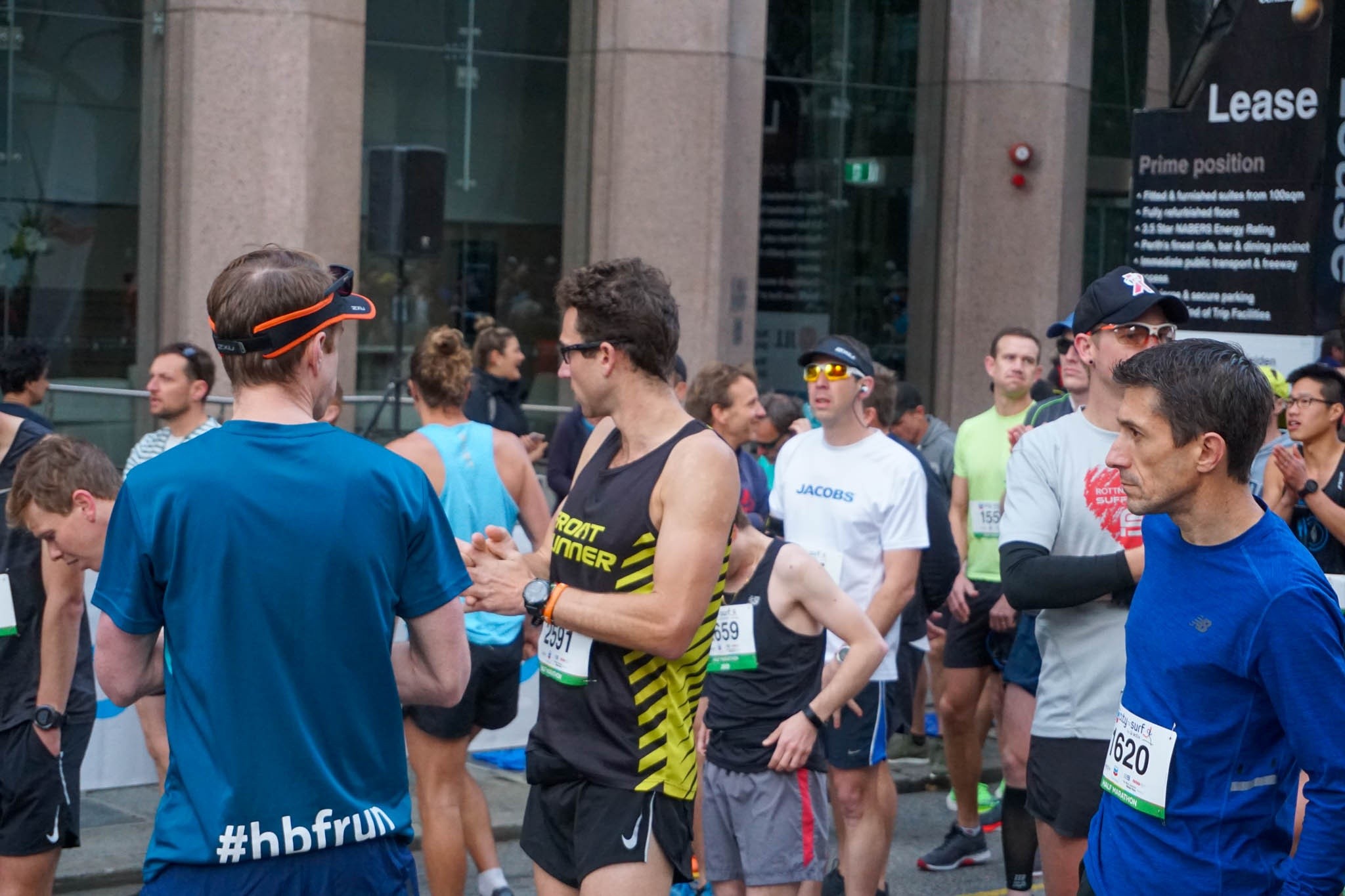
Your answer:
[522,577,551,626]
[33,704,67,731]
[1297,478,1320,500]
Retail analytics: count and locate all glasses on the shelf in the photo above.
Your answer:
[177,344,204,381]
[324,264,355,297]
[1088,322,1177,352]
[557,339,621,364]
[803,362,865,382]
[1057,339,1075,355]
[756,433,785,450]
[1280,393,1333,411]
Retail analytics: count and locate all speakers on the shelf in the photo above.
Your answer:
[368,145,445,258]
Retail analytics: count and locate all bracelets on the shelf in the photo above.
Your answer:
[801,705,825,730]
[543,578,571,628]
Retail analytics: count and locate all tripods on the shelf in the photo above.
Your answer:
[361,258,415,440]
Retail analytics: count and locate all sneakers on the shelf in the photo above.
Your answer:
[980,798,1004,831]
[930,741,951,778]
[946,777,1006,812]
[885,729,931,764]
[669,876,714,896]
[917,821,992,872]
[822,858,889,896]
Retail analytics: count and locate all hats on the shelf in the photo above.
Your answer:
[207,290,377,359]
[1257,365,1288,400]
[1072,264,1191,340]
[673,353,687,382]
[891,384,923,420]
[1047,310,1074,338]
[797,336,874,379]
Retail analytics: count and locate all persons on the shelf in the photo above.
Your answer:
[684,364,772,522]
[999,265,1189,896]
[6,433,169,800]
[385,323,552,895]
[665,355,689,403]
[1076,339,1344,896]
[1261,364,1344,575]
[1249,364,1304,498]
[767,340,932,896]
[885,384,953,790]
[90,243,474,895]
[459,258,741,896]
[464,325,550,464]
[546,398,610,517]
[695,508,888,896]
[919,328,1068,872]
[998,311,1091,896]
[121,341,224,796]
[313,380,344,426]
[751,392,814,490]
[821,358,962,896]
[0,338,93,895]
